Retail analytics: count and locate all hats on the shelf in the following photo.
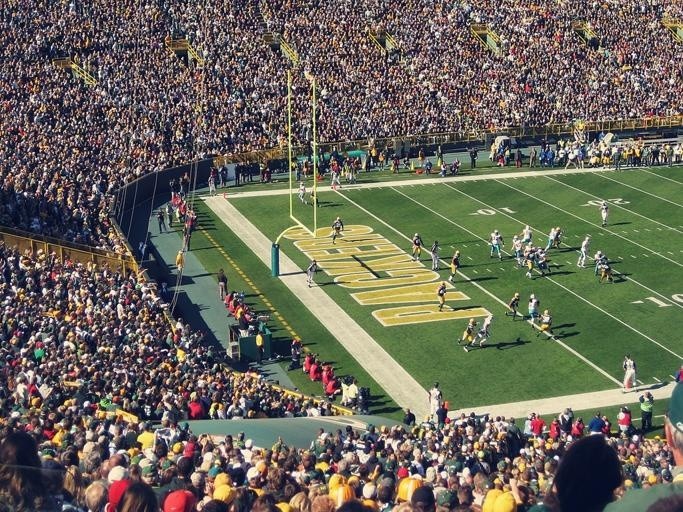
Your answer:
[213,473,235,504]
[107,465,132,504]
[173,442,185,453]
[397,476,424,501]
[163,489,194,512]
[329,473,359,506]
[482,489,517,512]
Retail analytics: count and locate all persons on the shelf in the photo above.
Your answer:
[2,1,682,511]
